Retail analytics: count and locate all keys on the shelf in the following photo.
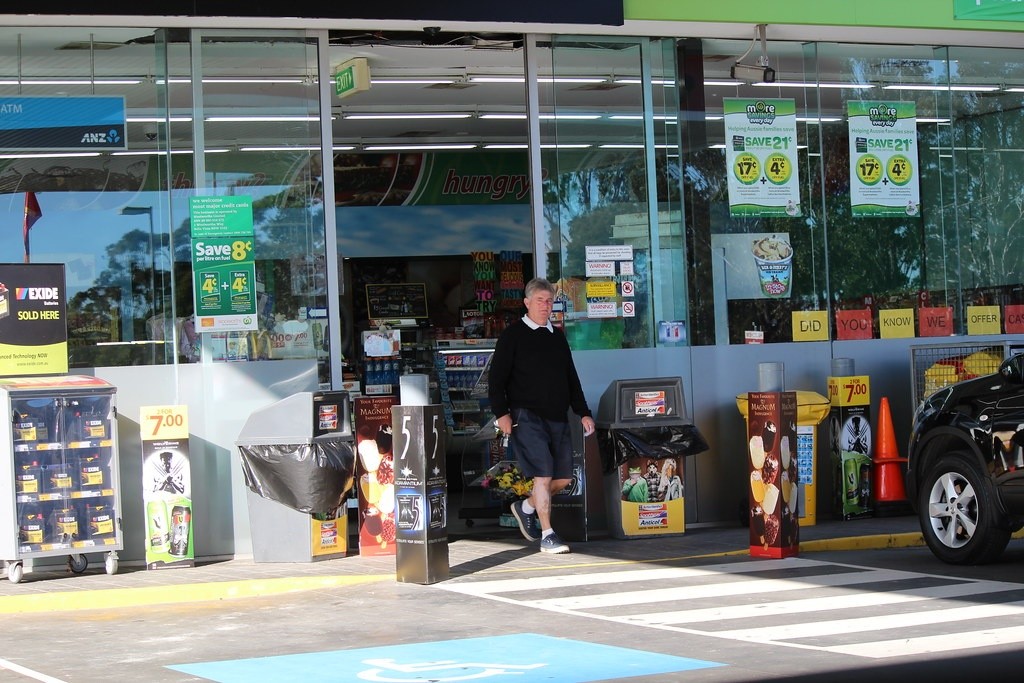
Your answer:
[493,418,519,448]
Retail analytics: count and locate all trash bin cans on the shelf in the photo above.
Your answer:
[595,375,696,540]
[230,389,356,564]
[736,386,829,528]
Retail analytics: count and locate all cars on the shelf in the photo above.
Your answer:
[908,353,1024,567]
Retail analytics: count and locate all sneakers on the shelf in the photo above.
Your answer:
[540,532,570,553]
[510,500,540,541]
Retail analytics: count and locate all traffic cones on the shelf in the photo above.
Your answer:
[874,397,912,517]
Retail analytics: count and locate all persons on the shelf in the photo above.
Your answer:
[154,451,185,495]
[487,278,596,556]
[847,416,869,453]
[145,262,196,364]
[831,418,840,456]
